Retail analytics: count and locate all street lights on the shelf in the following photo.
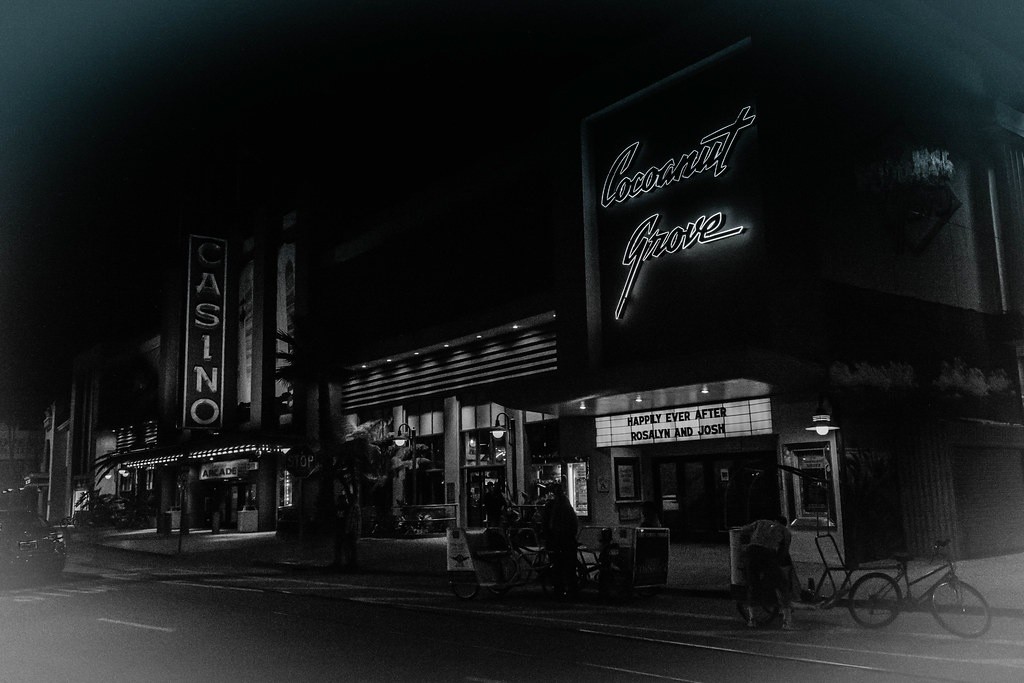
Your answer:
[806,402,846,568]
[396,422,418,522]
[490,411,519,505]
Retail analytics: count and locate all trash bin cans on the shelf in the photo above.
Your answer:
[166,507,182,529]
[237,505,259,533]
[156,513,172,537]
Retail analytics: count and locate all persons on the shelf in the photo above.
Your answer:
[741,514,795,630]
[482,482,508,523]
[539,483,578,605]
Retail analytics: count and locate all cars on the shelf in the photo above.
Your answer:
[0,510,67,595]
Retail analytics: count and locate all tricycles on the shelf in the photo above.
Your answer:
[437,524,668,603]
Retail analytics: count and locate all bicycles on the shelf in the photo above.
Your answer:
[848,538,993,641]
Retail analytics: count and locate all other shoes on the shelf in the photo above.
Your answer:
[748,621,756,628]
[783,622,803,631]
